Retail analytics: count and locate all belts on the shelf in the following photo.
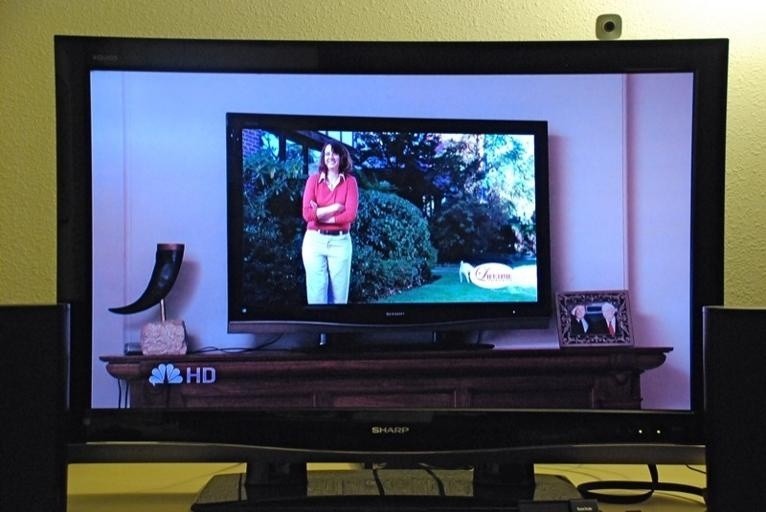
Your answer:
[320,229,348,235]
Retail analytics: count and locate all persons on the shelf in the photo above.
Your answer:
[591,302,620,333]
[300,140,360,304]
[571,304,592,334]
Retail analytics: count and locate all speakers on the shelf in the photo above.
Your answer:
[703,306,766,512]
[0,305,70,512]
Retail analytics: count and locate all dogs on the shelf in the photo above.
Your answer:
[459,260,474,284]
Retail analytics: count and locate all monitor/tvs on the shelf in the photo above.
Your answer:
[225,112,552,353]
[53,35,729,512]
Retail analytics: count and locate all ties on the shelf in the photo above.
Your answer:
[579,319,583,332]
[608,321,614,336]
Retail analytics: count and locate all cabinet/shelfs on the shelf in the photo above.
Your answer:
[99,345,674,410]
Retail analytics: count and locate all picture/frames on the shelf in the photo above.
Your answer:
[556,290,635,347]
